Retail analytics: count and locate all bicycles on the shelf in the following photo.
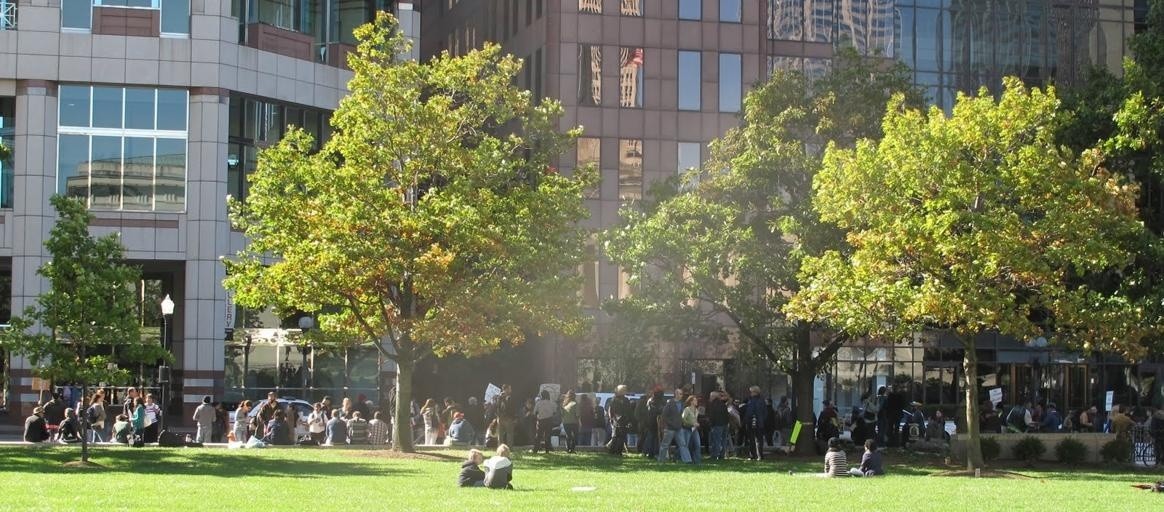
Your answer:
[1142,441,1164,468]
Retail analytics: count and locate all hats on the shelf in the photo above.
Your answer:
[34,407,43,414]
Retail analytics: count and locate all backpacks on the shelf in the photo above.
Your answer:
[87,407,100,424]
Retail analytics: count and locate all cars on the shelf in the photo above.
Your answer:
[1109,406,1158,443]
[221,396,347,443]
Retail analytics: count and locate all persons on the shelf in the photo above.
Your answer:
[817,386,965,477]
[977,401,1153,441]
[411,384,790,463]
[234,384,396,447]
[24,387,162,444]
[192,394,228,443]
[457,445,513,490]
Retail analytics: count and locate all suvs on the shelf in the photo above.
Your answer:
[841,407,956,442]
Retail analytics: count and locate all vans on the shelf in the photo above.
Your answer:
[534,389,640,450]
[612,392,743,446]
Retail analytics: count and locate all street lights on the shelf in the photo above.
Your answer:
[298,316,313,400]
[159,293,174,435]
